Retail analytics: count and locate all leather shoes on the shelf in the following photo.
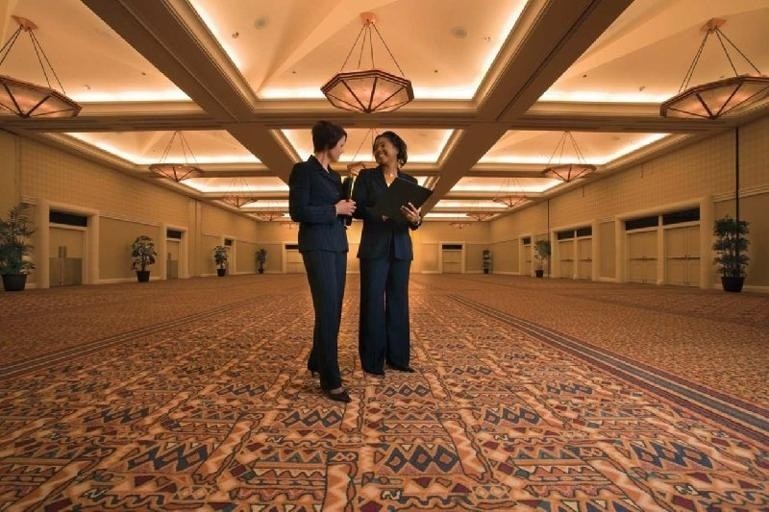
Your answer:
[398,366,415,373]
[327,389,350,402]
[370,369,385,375]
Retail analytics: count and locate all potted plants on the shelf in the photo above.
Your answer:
[481,251,491,273]
[0,202,40,292]
[532,240,548,277]
[711,216,752,293]
[256,249,266,273]
[213,245,228,277]
[131,235,158,283]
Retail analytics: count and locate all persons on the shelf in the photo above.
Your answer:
[288,119,358,403]
[351,131,423,376]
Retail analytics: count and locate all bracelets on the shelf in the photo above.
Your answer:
[410,220,421,227]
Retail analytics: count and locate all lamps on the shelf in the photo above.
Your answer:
[148,130,286,223]
[659,16,768,122]
[446,129,596,232]
[320,11,415,114]
[0,15,82,122]
